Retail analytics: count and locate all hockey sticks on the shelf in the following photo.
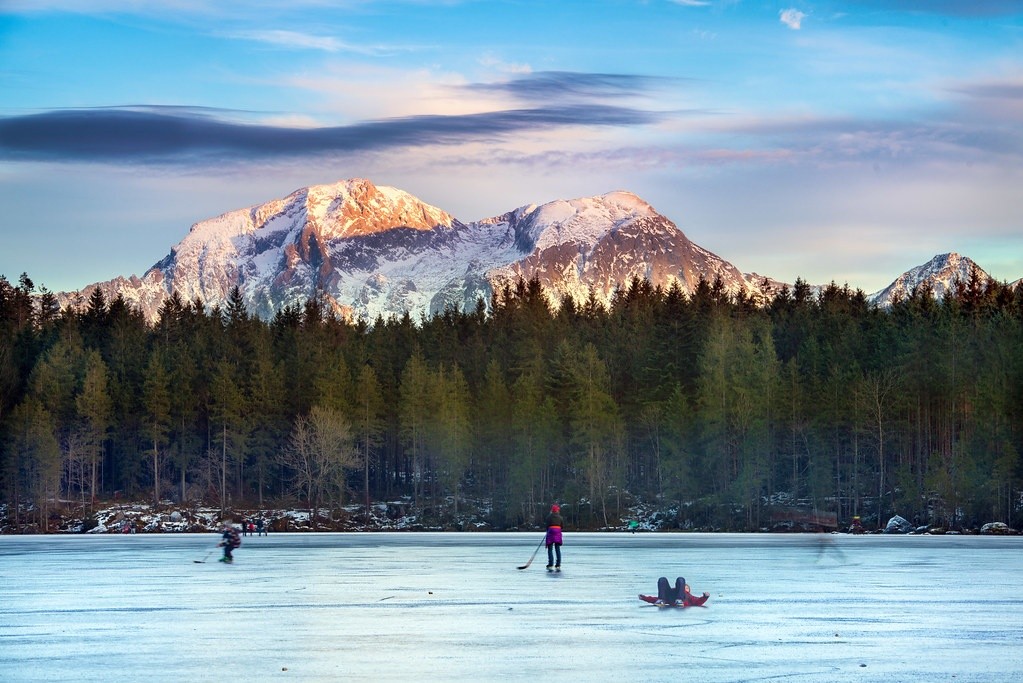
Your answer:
[194,544,224,564]
[517,532,549,571]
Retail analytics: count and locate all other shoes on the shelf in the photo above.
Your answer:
[546,565,553,570]
[556,566,561,571]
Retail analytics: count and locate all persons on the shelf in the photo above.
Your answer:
[243,519,247,537]
[545,504,563,570]
[262,518,270,536]
[630,517,639,533]
[249,523,255,535]
[216,524,241,563]
[256,517,263,536]
[637,576,711,608]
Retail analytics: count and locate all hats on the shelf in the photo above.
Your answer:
[552,505,559,512]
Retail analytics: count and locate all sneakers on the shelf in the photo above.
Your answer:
[654,599,665,605]
[675,599,684,607]
